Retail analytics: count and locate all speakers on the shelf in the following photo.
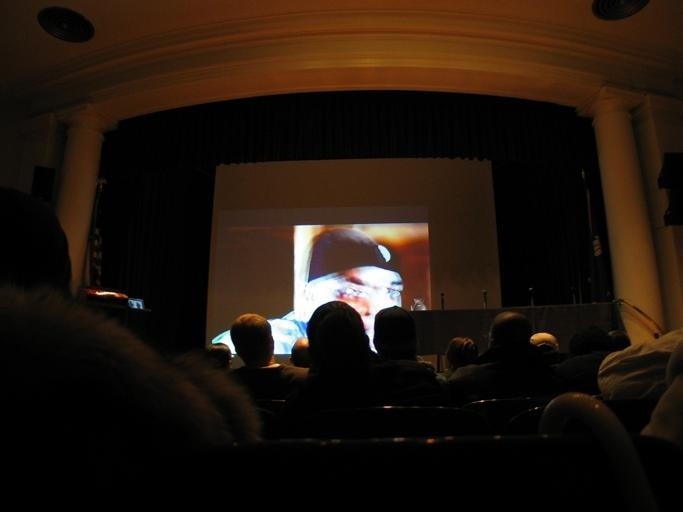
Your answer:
[657,151,683,225]
[29,165,56,202]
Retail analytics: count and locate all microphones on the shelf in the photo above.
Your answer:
[440,291,444,310]
[529,287,535,306]
[569,285,577,305]
[482,289,488,307]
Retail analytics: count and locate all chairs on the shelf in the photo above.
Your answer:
[181,396,681,509]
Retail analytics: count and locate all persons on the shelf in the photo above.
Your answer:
[0,184,261,443]
[211,228,403,354]
[205,302,632,406]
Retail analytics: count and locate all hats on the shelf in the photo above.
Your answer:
[308,229,401,281]
[530,333,557,347]
[375,307,416,350]
